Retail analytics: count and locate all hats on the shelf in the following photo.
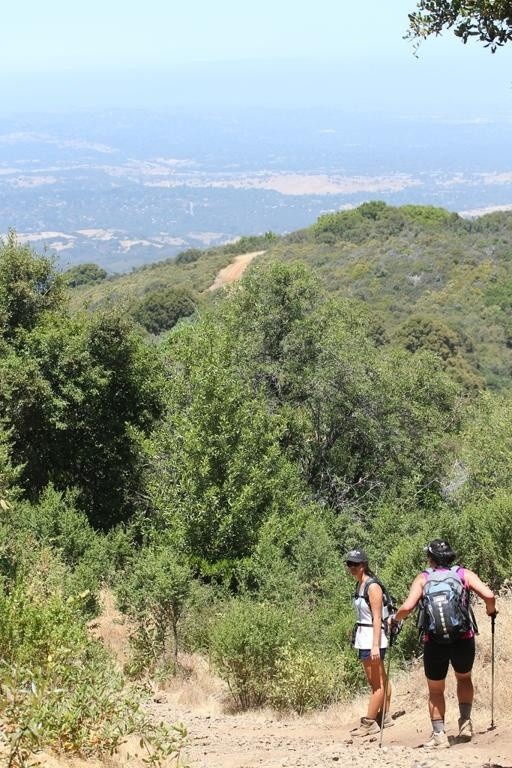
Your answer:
[423,538,451,561]
[343,549,367,564]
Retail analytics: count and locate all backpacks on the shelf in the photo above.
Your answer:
[417,563,479,649]
[364,578,403,637]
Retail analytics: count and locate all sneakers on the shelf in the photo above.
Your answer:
[350,717,381,737]
[458,718,474,740]
[422,731,451,749]
[376,712,395,728]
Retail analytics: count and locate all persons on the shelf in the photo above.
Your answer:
[387,539,495,748]
[344,548,393,738]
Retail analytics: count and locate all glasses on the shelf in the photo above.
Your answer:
[347,562,362,567]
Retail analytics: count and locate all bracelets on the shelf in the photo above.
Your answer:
[390,614,399,624]
